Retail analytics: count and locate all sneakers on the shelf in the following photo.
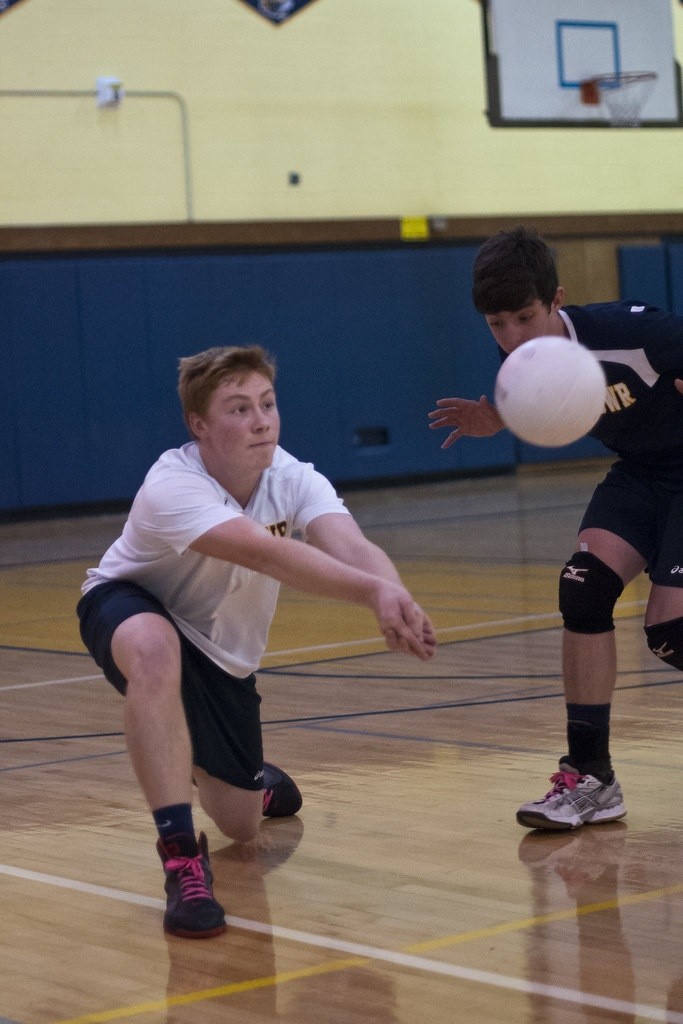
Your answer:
[516,756,628,831]
[155,829,229,939]
[191,762,303,816]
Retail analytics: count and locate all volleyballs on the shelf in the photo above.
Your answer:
[494,334,609,450]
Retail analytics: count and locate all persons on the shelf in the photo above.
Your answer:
[428,224,683,832]
[76,344,435,940]
